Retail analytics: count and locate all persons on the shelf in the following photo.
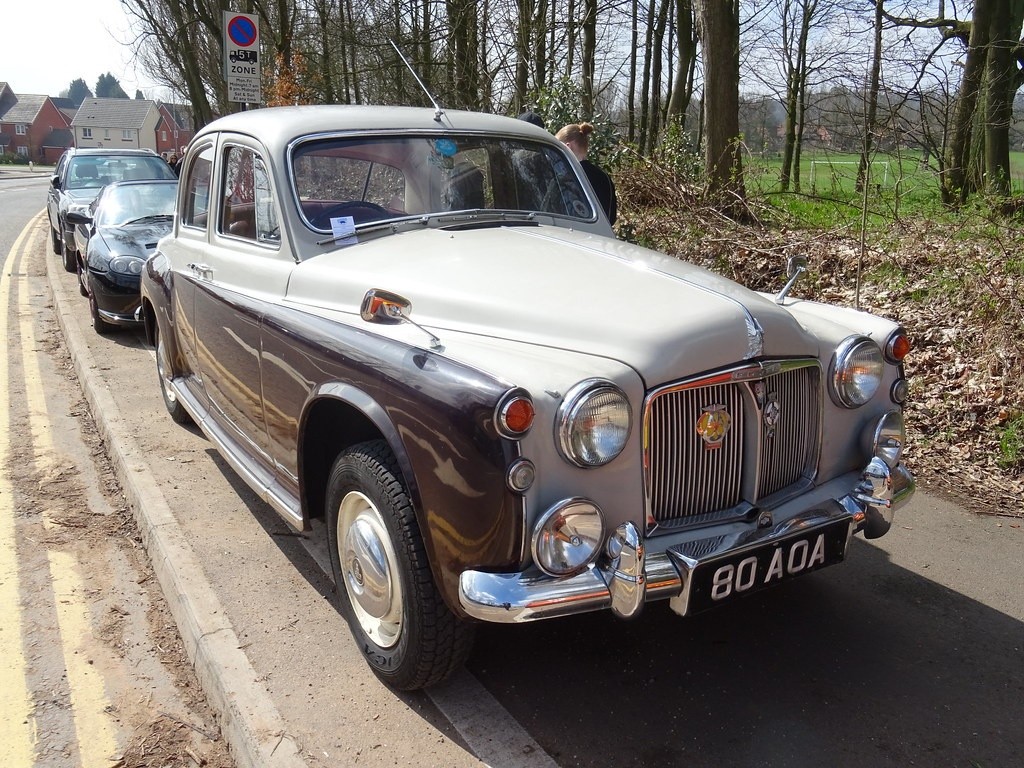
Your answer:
[161,145,187,179]
[539,124,617,227]
[510,112,554,210]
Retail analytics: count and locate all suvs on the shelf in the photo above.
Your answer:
[140,103,912,692]
[46,148,178,271]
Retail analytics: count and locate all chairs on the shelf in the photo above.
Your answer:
[123,168,147,178]
[111,188,153,224]
[71,165,103,186]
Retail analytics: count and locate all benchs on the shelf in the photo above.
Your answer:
[194,200,409,230]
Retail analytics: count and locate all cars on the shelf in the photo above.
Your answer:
[66,180,178,334]
